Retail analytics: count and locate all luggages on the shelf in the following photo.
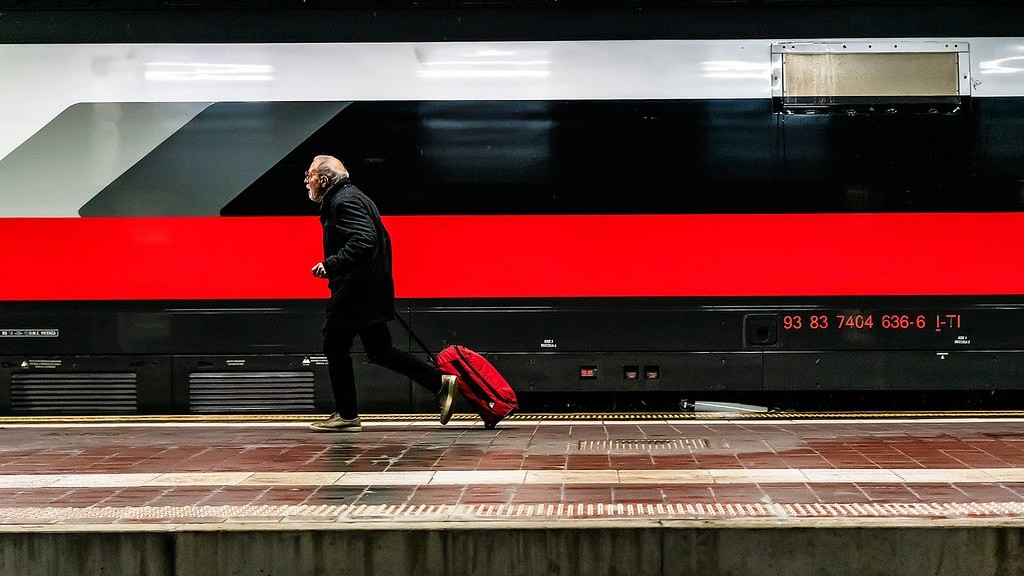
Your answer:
[394,307,521,429]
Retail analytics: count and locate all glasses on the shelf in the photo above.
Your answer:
[304,171,317,179]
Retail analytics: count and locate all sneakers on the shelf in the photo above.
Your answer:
[310,412,363,433]
[437,375,459,425]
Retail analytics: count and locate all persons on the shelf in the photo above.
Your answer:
[303,155,460,433]
